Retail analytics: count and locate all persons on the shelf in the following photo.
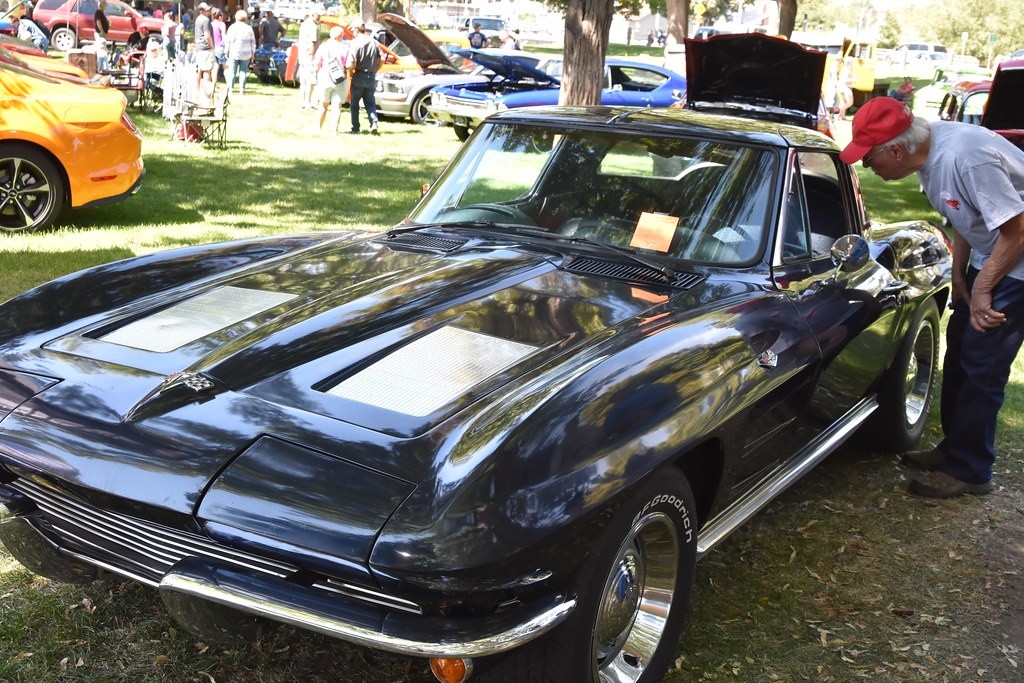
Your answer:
[112,0,286,95]
[298,8,325,110]
[345,20,385,134]
[20,15,51,39]
[8,15,48,54]
[0,0,9,12]
[498,29,520,50]
[824,56,856,120]
[647,29,654,46]
[627,27,632,44]
[839,97,1024,499]
[313,26,350,131]
[93,1,109,41]
[890,76,912,100]
[468,23,491,48]
[658,28,663,46]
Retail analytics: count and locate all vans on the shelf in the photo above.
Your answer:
[881,42,1024,72]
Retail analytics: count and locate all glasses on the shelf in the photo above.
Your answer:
[859,145,891,163]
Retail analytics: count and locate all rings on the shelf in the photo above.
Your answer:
[983,315,988,320]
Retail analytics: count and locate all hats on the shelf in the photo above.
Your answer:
[198,2,211,11]
[839,97,914,164]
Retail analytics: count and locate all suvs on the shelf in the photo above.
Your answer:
[456,12,522,50]
[32,0,185,56]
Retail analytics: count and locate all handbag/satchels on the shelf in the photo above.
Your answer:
[324,44,345,84]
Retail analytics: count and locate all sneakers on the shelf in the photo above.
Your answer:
[910,471,993,498]
[902,447,949,470]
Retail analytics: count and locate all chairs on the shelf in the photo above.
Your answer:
[166,87,229,147]
[100,52,147,114]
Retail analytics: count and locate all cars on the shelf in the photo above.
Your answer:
[666,28,835,136]
[1,104,955,683]
[939,61,1024,152]
[248,0,523,136]
[0,21,148,237]
[428,45,688,148]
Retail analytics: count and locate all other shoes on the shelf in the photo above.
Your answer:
[349,131,360,134]
[370,125,378,135]
